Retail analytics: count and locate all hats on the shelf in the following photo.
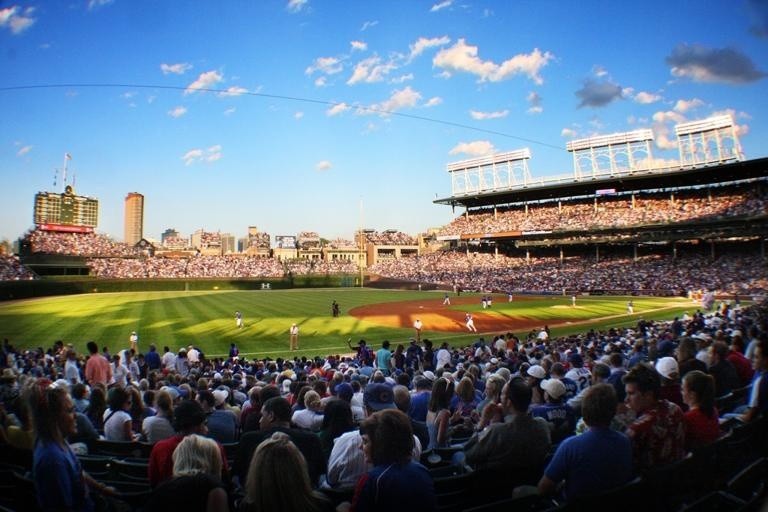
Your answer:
[335,338,436,411]
[656,356,680,381]
[165,349,290,405]
[729,330,742,340]
[488,345,583,400]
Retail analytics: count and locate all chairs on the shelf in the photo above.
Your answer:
[0,408,767,510]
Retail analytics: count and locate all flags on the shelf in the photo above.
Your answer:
[65,152,73,161]
